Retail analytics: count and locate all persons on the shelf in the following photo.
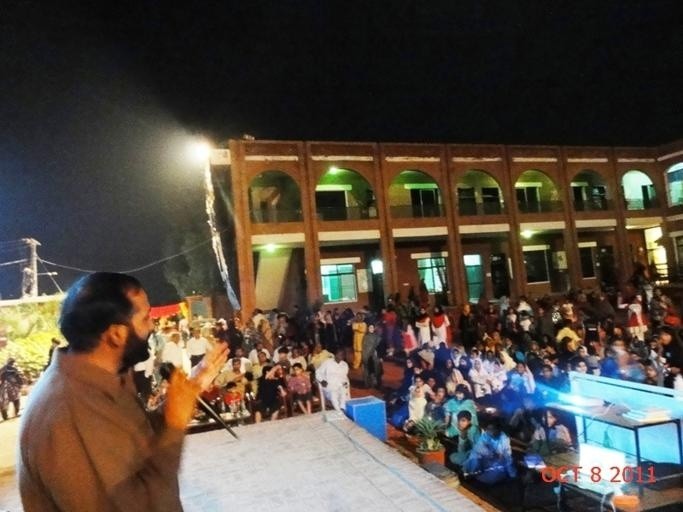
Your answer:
[0,272,682,511]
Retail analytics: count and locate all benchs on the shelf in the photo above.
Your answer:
[187,395,320,435]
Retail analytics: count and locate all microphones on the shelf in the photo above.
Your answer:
[159,361,239,439]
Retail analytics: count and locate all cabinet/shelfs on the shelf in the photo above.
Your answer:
[520,393,683,512]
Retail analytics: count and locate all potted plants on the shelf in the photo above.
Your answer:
[415,417,446,466]
